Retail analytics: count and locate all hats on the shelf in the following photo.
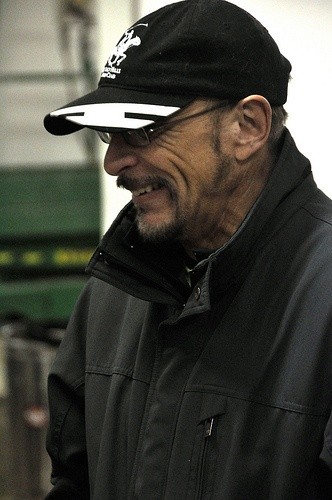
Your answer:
[43,0,288,136]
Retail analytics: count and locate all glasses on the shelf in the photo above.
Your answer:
[96,101,234,147]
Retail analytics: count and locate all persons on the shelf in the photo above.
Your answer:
[45,0,332,500]
